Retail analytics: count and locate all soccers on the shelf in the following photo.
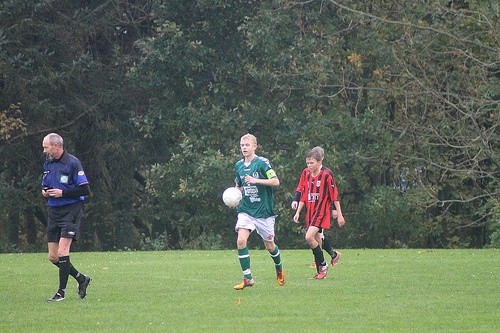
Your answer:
[222,186,243,207]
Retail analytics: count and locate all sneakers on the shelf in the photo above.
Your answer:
[276,266,286,286]
[78,276,92,298]
[314,262,328,279]
[311,261,316,267]
[331,252,341,267]
[47,292,66,302]
[234,278,254,290]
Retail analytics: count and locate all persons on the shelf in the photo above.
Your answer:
[291,146,345,280]
[228,134,286,291]
[41,132,92,302]
